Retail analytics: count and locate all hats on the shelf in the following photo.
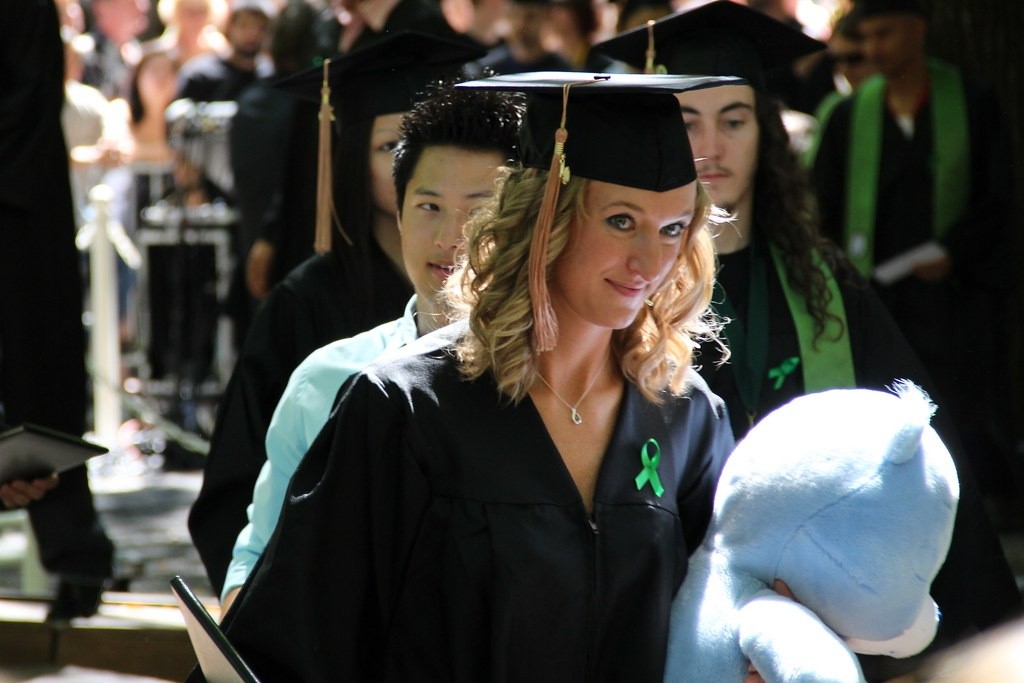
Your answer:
[592,0,828,96]
[850,0,932,19]
[271,33,488,257]
[453,71,749,349]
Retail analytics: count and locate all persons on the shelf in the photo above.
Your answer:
[0,0,1023,683]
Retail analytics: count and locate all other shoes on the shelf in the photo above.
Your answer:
[167,391,200,434]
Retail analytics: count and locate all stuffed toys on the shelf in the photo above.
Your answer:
[659,379,963,683]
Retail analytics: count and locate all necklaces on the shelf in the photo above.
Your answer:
[534,347,614,425]
